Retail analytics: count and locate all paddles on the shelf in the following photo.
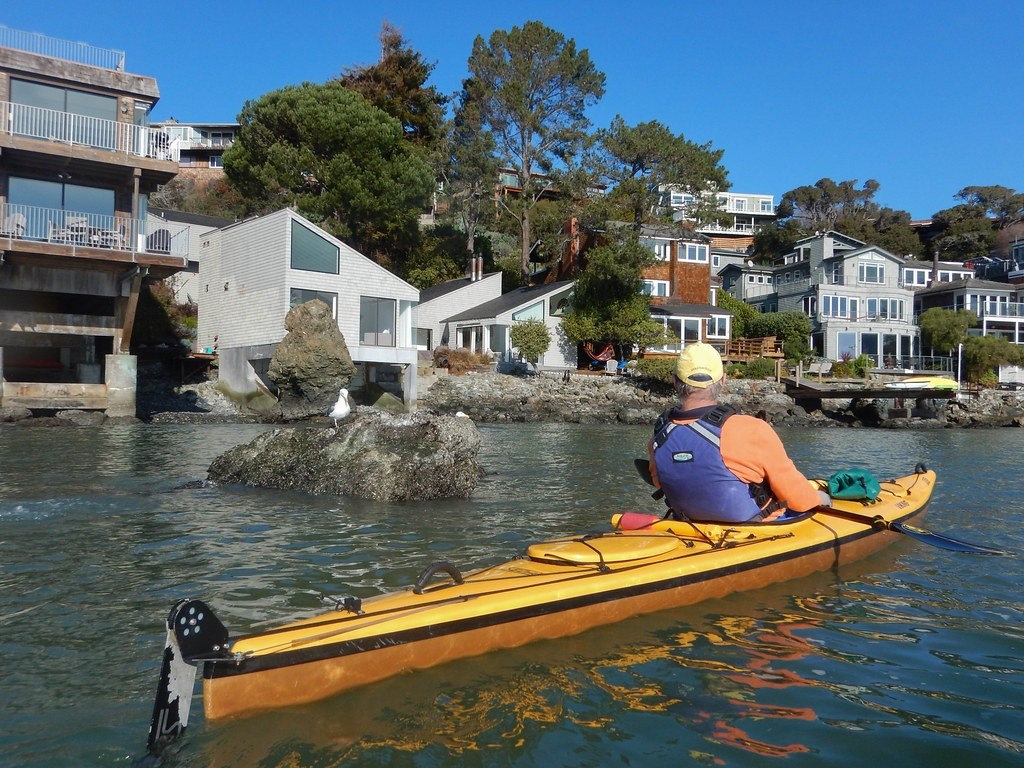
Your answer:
[626,457,1019,563]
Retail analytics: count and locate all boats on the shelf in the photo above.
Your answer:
[142,465,941,758]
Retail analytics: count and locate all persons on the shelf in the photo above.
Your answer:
[647,343,831,522]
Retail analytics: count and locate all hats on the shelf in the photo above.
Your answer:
[674,341,726,388]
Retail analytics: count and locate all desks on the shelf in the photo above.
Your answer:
[69,225,107,248]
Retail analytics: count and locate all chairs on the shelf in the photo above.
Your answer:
[0,212,126,251]
[803,362,833,375]
[604,359,619,376]
